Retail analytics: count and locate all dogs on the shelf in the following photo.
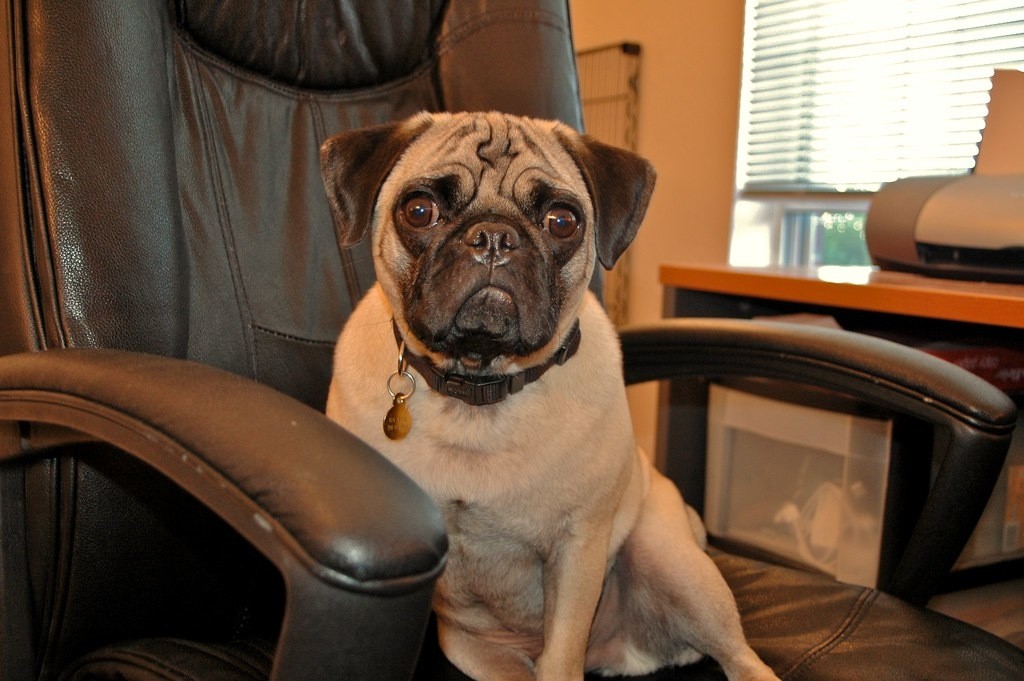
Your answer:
[318,112,781,681]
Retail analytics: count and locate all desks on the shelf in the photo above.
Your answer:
[647,258,1024,605]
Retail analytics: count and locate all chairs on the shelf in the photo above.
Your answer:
[2,0,1024,681]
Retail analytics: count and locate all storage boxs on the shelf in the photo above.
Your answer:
[697,371,1023,600]
